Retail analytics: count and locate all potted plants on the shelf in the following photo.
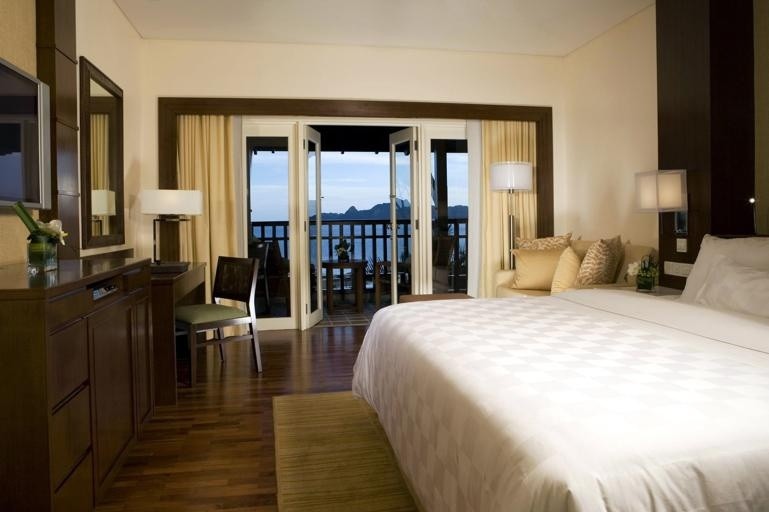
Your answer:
[12,201,68,272]
[335,239,350,263]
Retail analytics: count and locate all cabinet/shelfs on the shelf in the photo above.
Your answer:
[0,257,152,511]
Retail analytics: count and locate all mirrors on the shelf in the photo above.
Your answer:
[79,56,125,249]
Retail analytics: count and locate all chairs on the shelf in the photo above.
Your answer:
[175,256,262,387]
[254,242,270,305]
[433,235,457,283]
[494,239,658,298]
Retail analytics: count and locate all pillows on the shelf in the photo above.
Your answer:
[510,234,621,295]
[681,233,769,319]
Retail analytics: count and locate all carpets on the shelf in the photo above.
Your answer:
[272,391,418,512]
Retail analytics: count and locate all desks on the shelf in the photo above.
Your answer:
[152,261,207,406]
[323,259,367,315]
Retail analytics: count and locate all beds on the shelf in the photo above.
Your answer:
[352,234,769,512]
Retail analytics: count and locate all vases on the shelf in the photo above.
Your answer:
[636,275,655,292]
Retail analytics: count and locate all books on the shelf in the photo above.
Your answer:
[92,282,118,301]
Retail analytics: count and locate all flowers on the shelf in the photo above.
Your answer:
[627,254,657,289]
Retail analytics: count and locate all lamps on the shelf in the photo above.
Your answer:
[489,161,533,270]
[140,190,204,262]
[633,169,688,233]
[91,189,116,217]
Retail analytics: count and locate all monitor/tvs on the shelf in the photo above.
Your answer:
[0,56,52,210]
